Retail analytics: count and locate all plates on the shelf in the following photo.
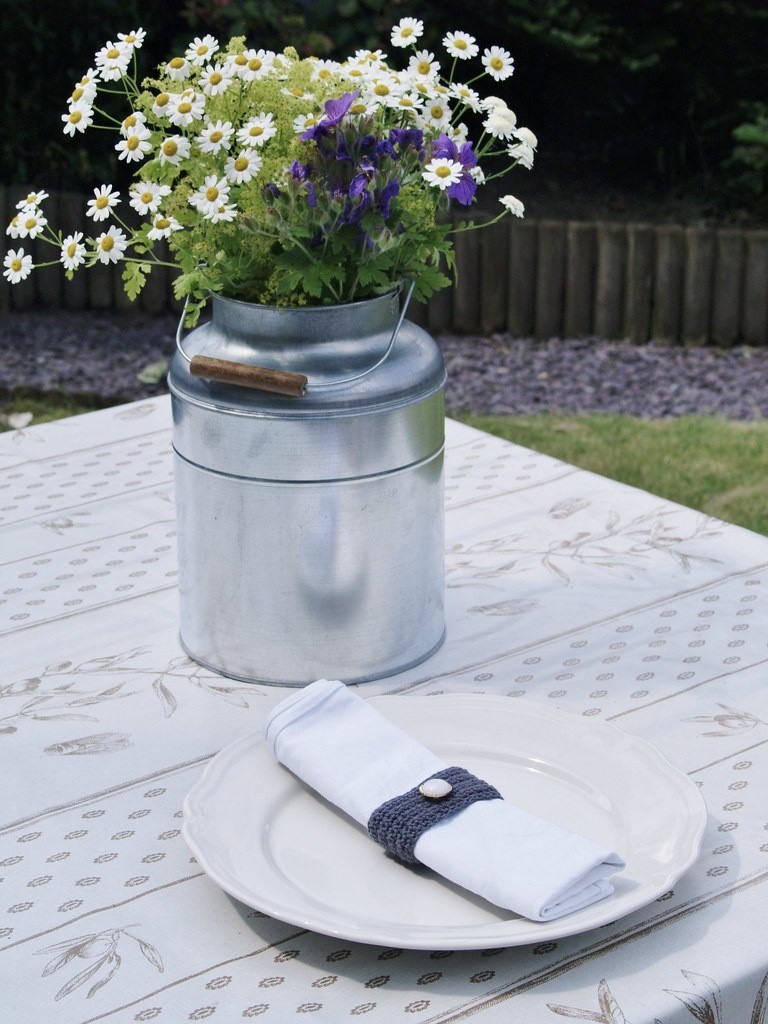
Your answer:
[181,692,708,951]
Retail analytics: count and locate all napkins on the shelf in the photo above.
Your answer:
[262,676,625,924]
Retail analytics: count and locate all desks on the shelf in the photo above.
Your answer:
[0,393,768,1024]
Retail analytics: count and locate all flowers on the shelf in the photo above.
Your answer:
[0,18,539,331]
